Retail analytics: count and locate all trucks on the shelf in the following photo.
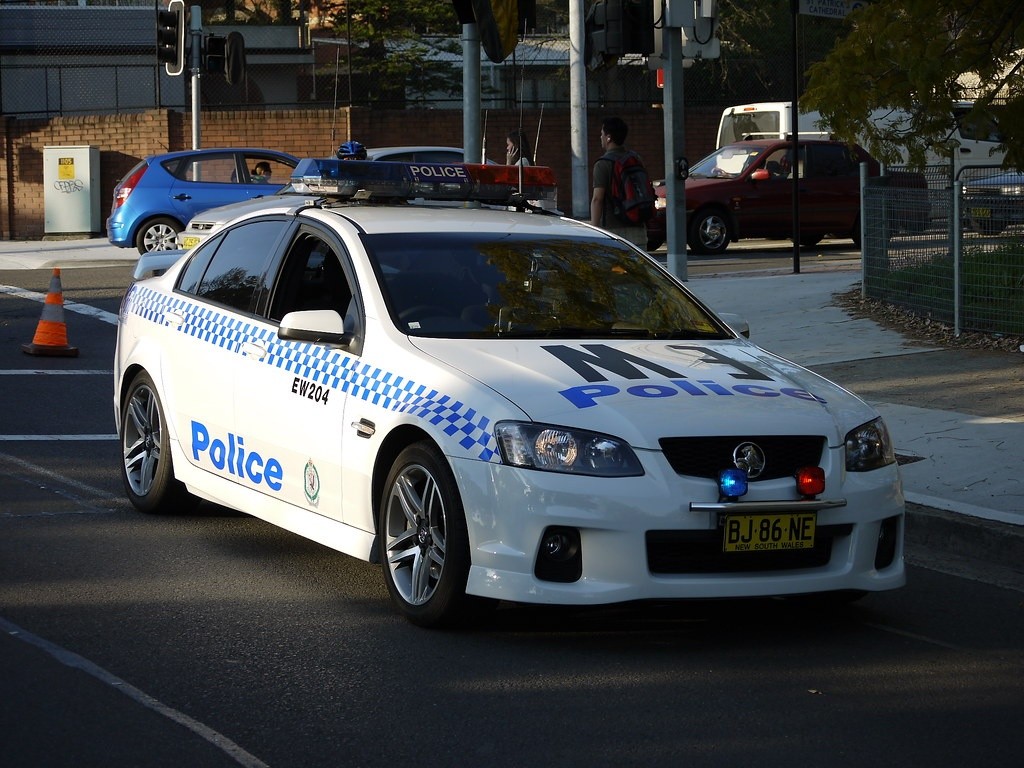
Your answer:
[713,96,1015,215]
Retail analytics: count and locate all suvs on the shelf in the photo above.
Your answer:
[642,130,930,253]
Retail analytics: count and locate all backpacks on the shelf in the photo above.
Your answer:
[593,146,658,223]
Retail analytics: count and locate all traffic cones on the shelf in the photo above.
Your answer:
[23,269,80,358]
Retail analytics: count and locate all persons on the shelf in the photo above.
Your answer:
[590,118,648,253]
[336,141,367,161]
[505,129,542,213]
[770,154,791,180]
[251,162,272,185]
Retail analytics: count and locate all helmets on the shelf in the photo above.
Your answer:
[336,140,368,160]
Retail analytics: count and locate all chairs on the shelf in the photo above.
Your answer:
[230,168,237,184]
[787,162,804,179]
[301,250,351,320]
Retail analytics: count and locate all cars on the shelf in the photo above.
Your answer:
[107,148,299,257]
[964,170,1024,234]
[360,145,503,208]
[101,155,906,626]
